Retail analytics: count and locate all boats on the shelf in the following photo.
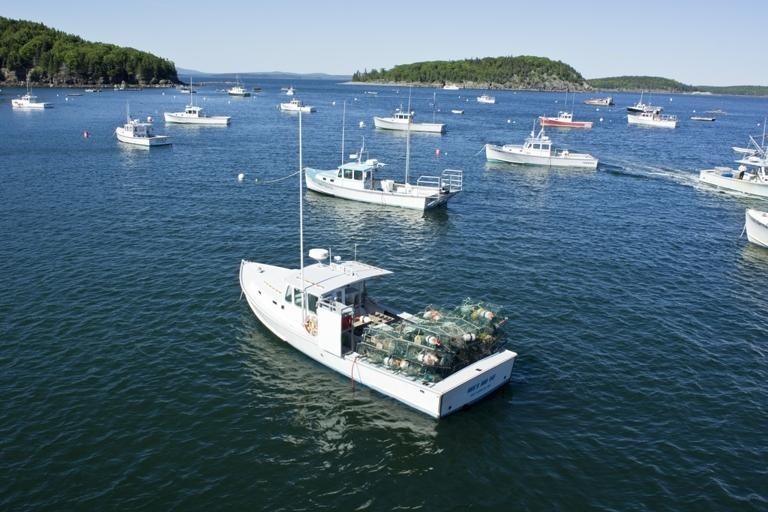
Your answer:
[10,75,55,111]
[232,106,523,419]
[371,90,448,136]
[172,78,302,97]
[698,117,767,202]
[163,76,233,127]
[275,98,315,114]
[113,99,173,150]
[737,206,768,251]
[482,112,603,171]
[442,76,716,130]
[304,78,463,210]
